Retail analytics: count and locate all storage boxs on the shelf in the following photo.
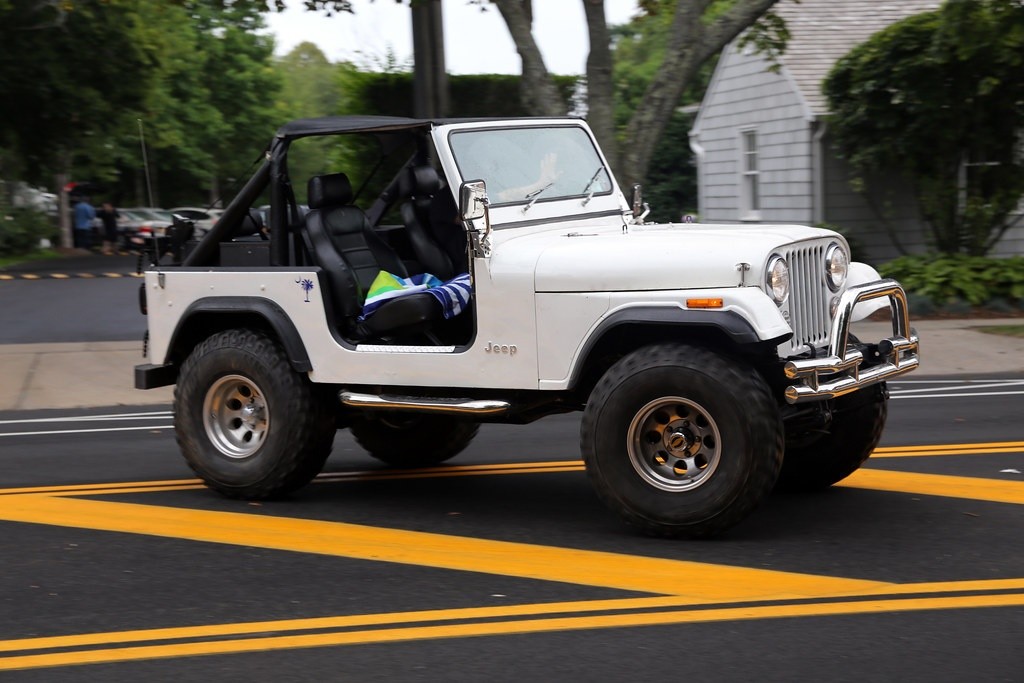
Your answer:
[187,235,273,267]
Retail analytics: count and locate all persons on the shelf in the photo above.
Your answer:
[71,195,95,251]
[96,200,120,255]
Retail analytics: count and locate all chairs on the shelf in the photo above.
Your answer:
[265,205,320,267]
[237,210,264,236]
[399,164,471,282]
[306,172,472,346]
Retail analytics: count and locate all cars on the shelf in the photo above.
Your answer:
[16,183,311,250]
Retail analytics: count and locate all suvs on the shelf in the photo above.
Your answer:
[131,112,922,542]
